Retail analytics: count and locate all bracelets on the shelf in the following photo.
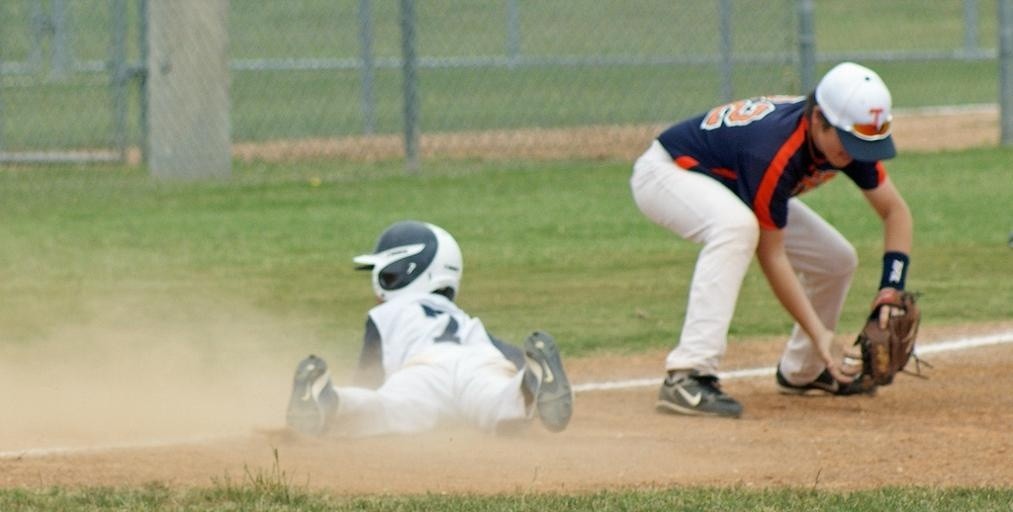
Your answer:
[878,249,910,289]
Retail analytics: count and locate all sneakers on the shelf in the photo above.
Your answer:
[775,363,854,395]
[287,356,332,438]
[524,332,573,434]
[657,375,741,418]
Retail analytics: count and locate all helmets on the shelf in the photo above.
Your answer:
[354,220,464,298]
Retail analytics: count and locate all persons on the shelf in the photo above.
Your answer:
[287,220,574,439]
[629,60,916,419]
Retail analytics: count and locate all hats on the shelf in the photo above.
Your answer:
[814,62,896,162]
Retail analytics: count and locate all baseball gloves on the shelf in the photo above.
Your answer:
[841,288,933,394]
